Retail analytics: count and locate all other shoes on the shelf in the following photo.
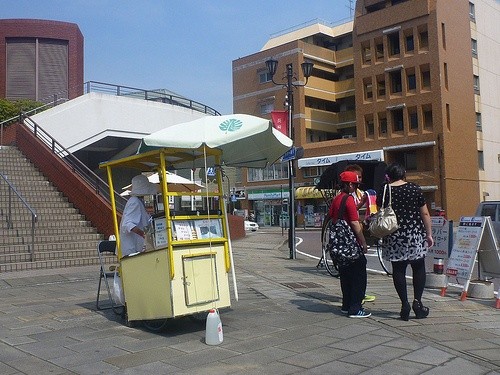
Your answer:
[348,310,372,318]
[363,295,375,301]
[341,307,365,313]
[361,300,366,305]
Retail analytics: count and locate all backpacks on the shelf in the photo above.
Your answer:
[326,220,364,269]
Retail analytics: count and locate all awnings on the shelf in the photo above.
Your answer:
[295,186,340,199]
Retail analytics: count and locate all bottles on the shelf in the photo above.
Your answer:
[205,309,224,346]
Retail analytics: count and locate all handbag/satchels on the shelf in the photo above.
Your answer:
[112,267,126,306]
[368,183,399,239]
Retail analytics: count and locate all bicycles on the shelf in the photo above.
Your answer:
[315,212,393,277]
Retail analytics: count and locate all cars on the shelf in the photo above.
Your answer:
[243,219,260,232]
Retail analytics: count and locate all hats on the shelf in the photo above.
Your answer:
[337,171,363,184]
[128,175,160,197]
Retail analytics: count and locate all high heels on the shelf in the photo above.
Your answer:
[399,302,411,321]
[412,300,430,318]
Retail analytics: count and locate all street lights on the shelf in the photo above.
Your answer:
[265,56,314,259]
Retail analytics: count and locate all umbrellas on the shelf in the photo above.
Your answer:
[110,113,293,308]
[120,170,207,215]
[317,160,389,193]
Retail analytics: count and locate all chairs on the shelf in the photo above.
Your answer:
[96,240,124,316]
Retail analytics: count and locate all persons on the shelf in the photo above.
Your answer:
[342,163,376,304]
[377,164,434,321]
[114,176,159,320]
[328,172,371,318]
